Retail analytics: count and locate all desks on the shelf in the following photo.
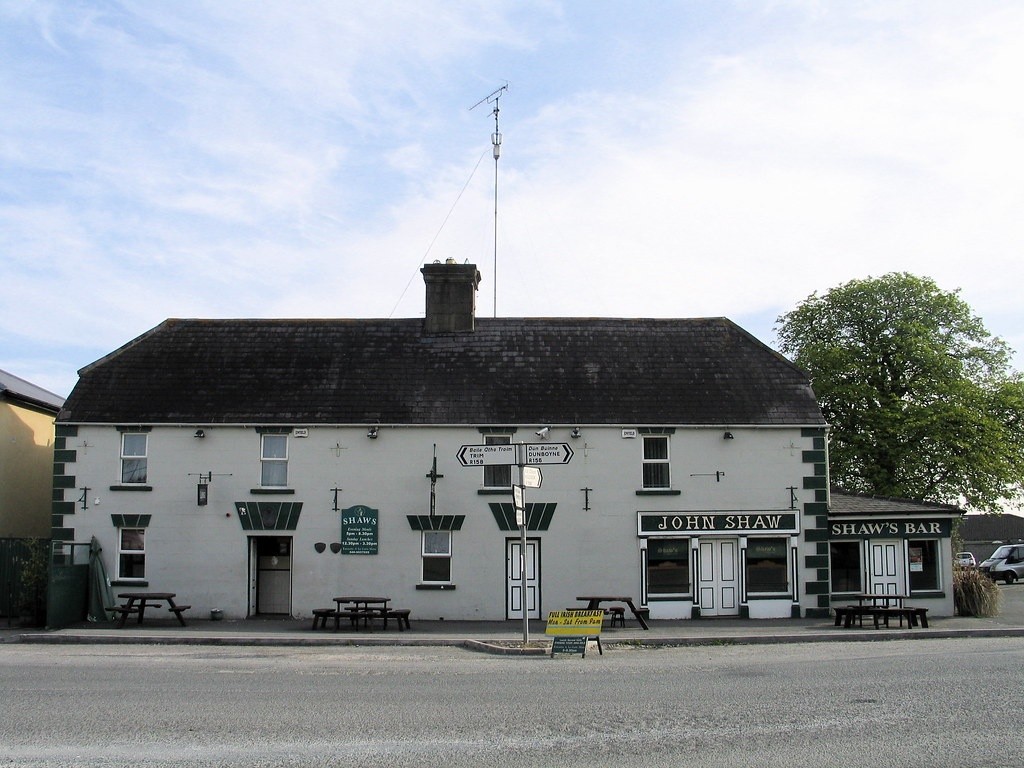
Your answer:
[311,609,335,614]
[117,593,185,629]
[332,597,391,630]
[855,595,906,628]
[576,596,649,630]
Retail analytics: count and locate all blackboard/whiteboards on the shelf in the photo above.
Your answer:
[551,636,588,653]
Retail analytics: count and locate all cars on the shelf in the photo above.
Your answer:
[954,552,976,569]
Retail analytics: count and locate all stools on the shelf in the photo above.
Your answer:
[609,606,626,627]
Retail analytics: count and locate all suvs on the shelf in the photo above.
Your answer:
[979,544,1024,584]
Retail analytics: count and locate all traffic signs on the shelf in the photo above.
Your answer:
[456,442,574,467]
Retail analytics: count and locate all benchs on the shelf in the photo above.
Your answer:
[120,604,162,608]
[908,606,928,613]
[632,608,648,614]
[832,606,857,614]
[858,608,914,616]
[106,607,138,614]
[847,604,888,609]
[565,608,608,611]
[332,612,385,619]
[344,607,390,611]
[388,609,410,618]
[167,606,191,612]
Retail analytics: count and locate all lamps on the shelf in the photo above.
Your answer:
[368,430,377,437]
[724,432,733,440]
[571,428,582,437]
[94,496,100,504]
[194,430,204,437]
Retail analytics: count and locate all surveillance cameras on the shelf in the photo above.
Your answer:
[536,427,548,436]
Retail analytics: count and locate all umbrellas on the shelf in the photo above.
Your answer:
[88,535,116,624]
